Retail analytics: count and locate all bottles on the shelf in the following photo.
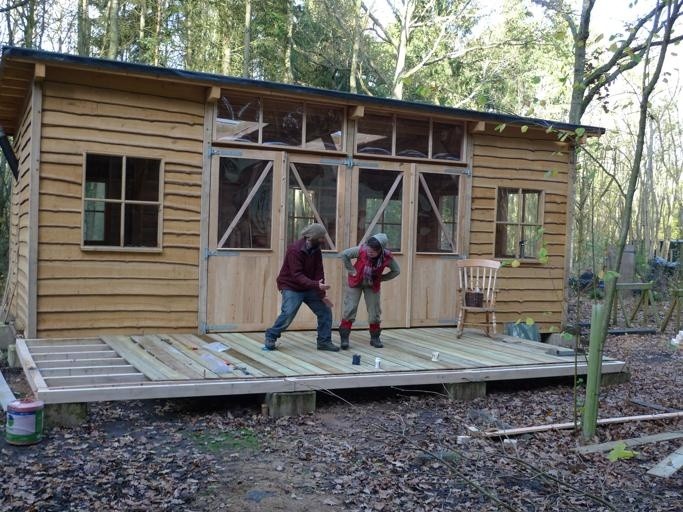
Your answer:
[374,357,381,369]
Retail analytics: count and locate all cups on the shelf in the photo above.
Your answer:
[431,352,440,361]
[351,354,361,365]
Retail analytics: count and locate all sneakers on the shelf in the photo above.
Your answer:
[317,342,338,351]
[266,339,275,350]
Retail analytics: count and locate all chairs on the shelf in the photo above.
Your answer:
[455,258,501,340]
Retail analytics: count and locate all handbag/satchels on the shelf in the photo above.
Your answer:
[465,292,483,307]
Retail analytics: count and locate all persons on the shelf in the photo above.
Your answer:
[579,267,593,288]
[264,223,340,352]
[338,232,401,348]
[599,266,607,277]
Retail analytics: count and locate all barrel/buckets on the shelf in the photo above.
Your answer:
[604,244,635,299]
[5,400,46,445]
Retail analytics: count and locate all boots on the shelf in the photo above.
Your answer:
[339,327,350,347]
[370,328,383,348]
